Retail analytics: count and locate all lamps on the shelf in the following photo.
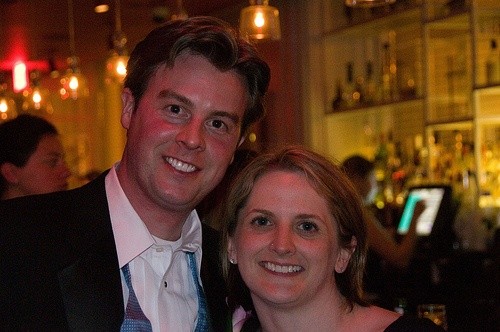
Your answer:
[59,0,90,101]
[239,0,282,43]
[104,1,132,86]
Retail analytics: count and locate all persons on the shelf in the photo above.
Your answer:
[0,16,271,332]
[195,148,260,232]
[340,154,427,269]
[0,112,72,200]
[218,143,445,332]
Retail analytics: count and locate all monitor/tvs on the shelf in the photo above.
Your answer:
[396,184,452,238]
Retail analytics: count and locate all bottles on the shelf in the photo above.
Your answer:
[486,37,500,85]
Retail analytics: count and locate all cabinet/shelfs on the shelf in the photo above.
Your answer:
[299,0,500,227]
